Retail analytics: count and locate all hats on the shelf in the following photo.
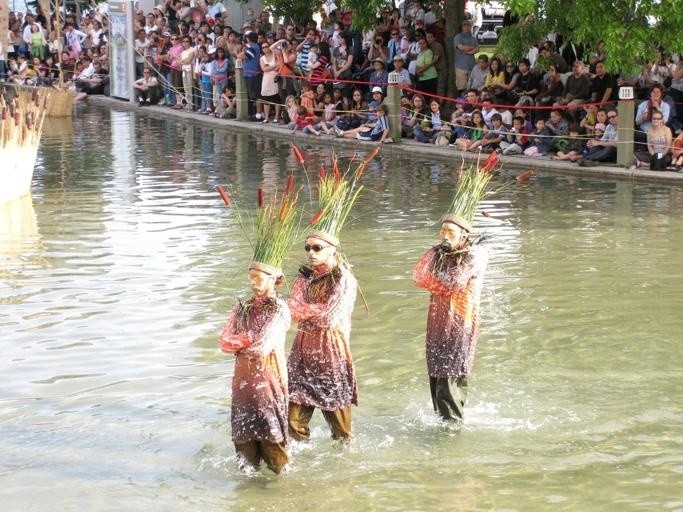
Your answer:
[147,5,173,38]
[372,55,404,65]
[371,86,384,93]
[240,22,255,38]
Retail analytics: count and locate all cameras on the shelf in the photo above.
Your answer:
[512,128,515,131]
[376,39,381,44]
[310,37,316,41]
[281,42,286,47]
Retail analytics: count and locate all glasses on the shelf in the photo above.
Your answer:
[304,245,331,252]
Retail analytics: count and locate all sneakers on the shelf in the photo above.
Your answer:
[578,158,601,167]
[663,162,682,172]
[138,98,344,139]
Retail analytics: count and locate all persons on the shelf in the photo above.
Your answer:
[411,223,488,420]
[285,237,358,443]
[219,269,291,475]
